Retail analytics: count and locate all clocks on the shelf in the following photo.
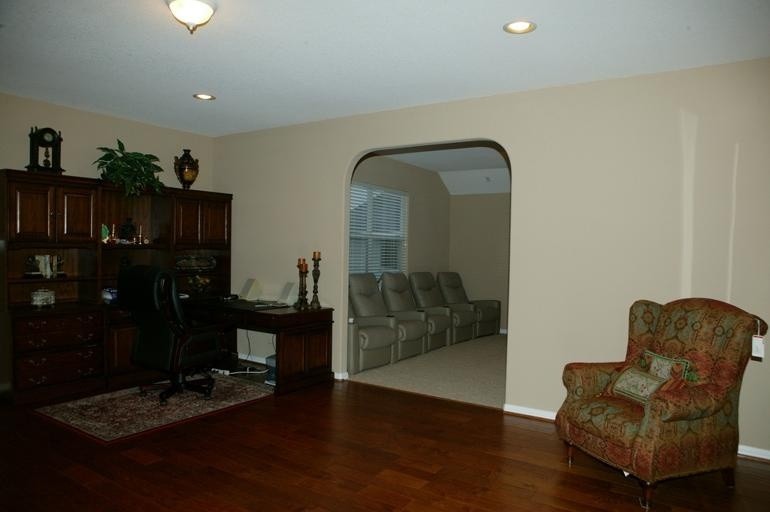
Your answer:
[25,125,66,175]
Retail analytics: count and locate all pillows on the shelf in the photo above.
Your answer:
[639,347,689,382]
[601,366,688,408]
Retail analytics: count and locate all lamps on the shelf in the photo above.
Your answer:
[160,2,220,36]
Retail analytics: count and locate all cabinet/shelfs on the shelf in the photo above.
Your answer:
[0,168,233,392]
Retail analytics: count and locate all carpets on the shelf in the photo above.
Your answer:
[31,371,277,445]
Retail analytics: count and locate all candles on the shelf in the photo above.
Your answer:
[296,251,320,272]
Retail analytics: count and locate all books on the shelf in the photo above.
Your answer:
[35,254,64,279]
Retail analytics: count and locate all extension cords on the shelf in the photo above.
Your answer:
[211,368,230,376]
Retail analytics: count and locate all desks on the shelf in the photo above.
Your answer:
[207,297,334,396]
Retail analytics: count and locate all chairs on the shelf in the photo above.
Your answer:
[554,298,768,512]
[347,272,500,374]
[117,265,219,404]
